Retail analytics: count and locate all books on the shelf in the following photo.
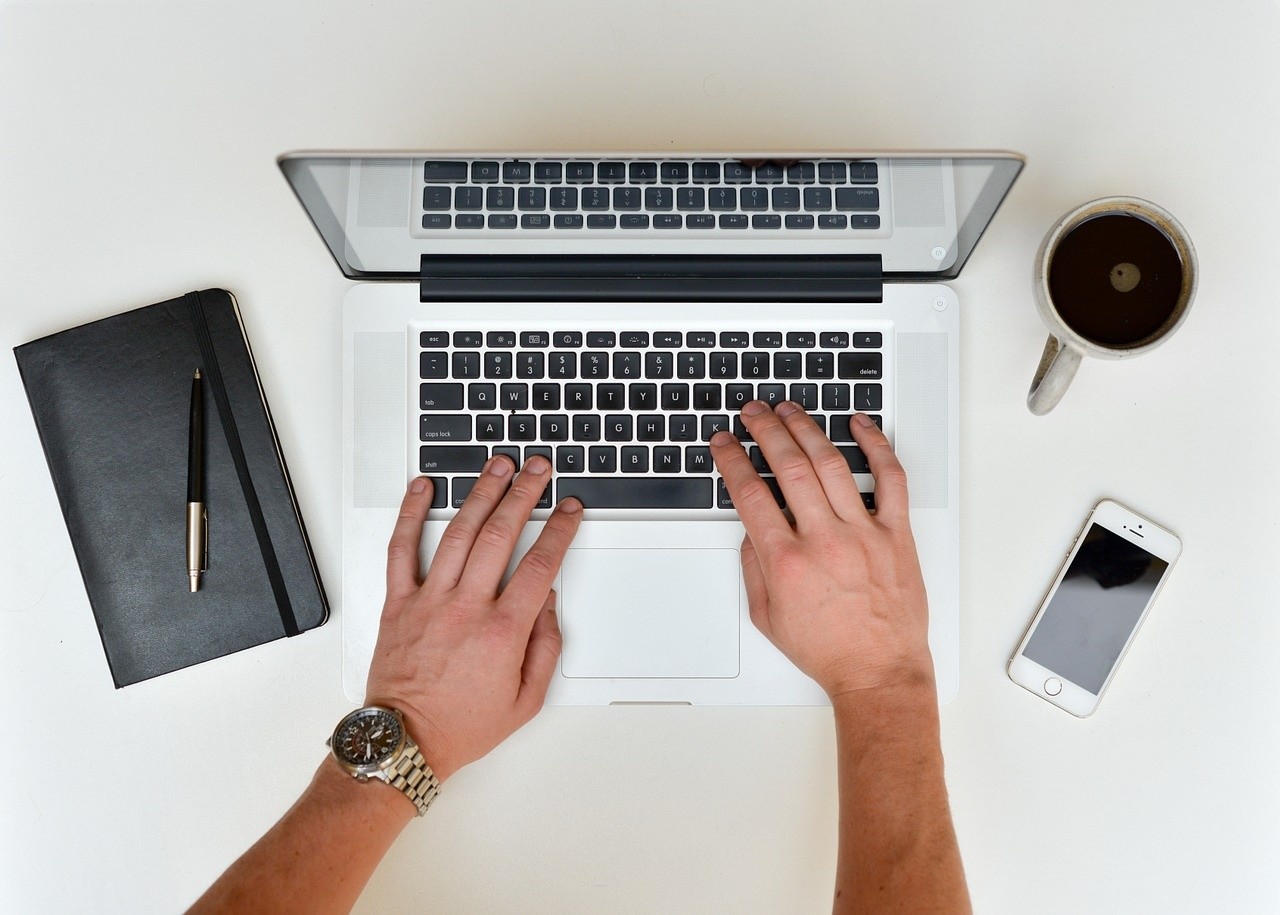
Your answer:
[9,287,328,688]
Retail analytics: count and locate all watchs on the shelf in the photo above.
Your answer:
[326,703,441,819]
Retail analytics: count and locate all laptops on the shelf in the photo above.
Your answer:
[269,144,1029,720]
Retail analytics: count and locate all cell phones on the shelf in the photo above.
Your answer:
[1005,496,1183,720]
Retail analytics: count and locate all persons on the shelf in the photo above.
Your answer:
[185,400,977,914]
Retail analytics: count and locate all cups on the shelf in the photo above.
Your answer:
[1029,193,1197,419]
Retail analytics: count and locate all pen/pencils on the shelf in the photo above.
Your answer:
[184,367,209,592]
